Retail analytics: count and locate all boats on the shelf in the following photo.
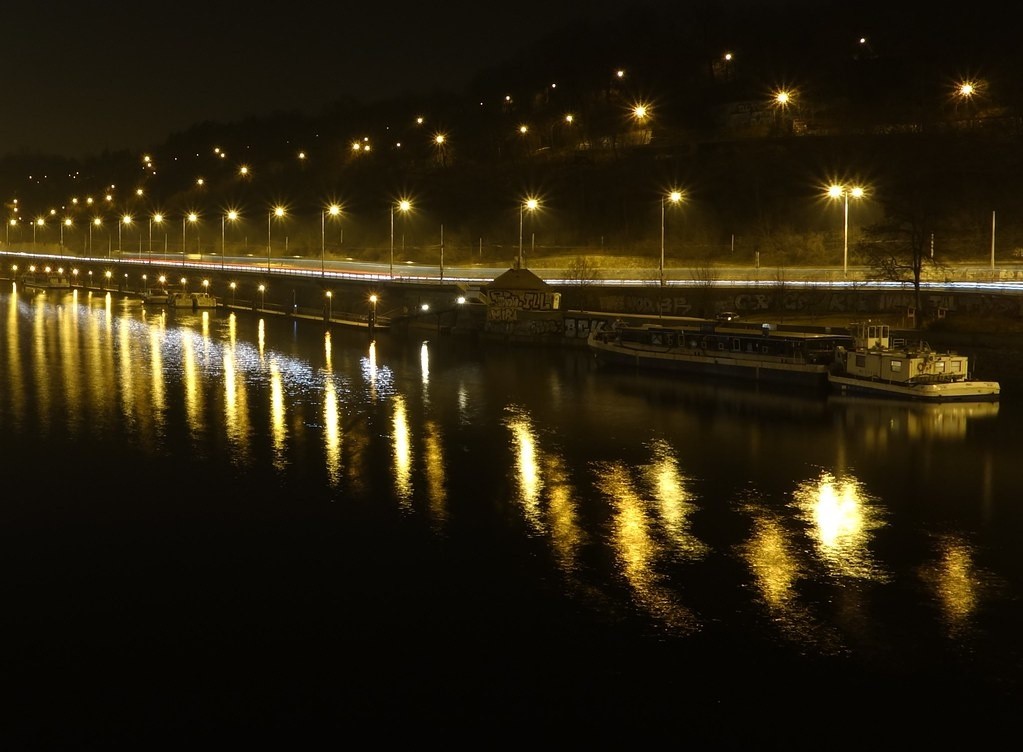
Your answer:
[586,321,999,401]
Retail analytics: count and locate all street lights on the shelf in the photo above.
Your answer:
[148,214,163,263]
[34,219,46,253]
[369,295,378,321]
[61,220,71,255]
[319,203,341,276]
[182,215,197,268]
[518,196,538,269]
[267,206,284,276]
[6,219,16,250]
[660,188,683,288]
[830,183,867,277]
[220,210,239,268]
[11,264,266,313]
[89,219,102,258]
[325,291,333,318]
[118,216,131,260]
[389,199,411,278]
